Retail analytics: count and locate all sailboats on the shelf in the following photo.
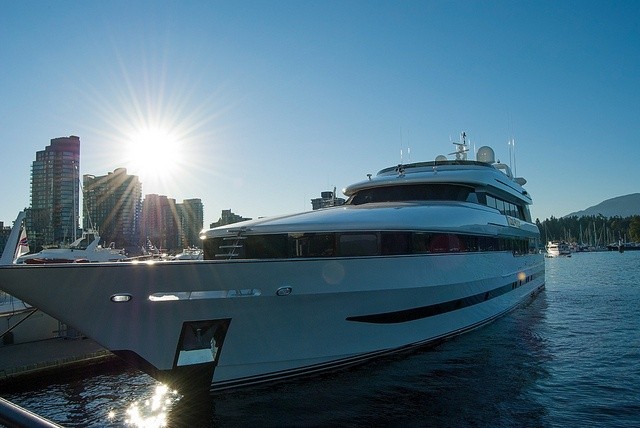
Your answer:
[15,159,129,263]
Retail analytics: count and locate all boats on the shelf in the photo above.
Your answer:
[546,240,573,258]
[0,130,547,401]
